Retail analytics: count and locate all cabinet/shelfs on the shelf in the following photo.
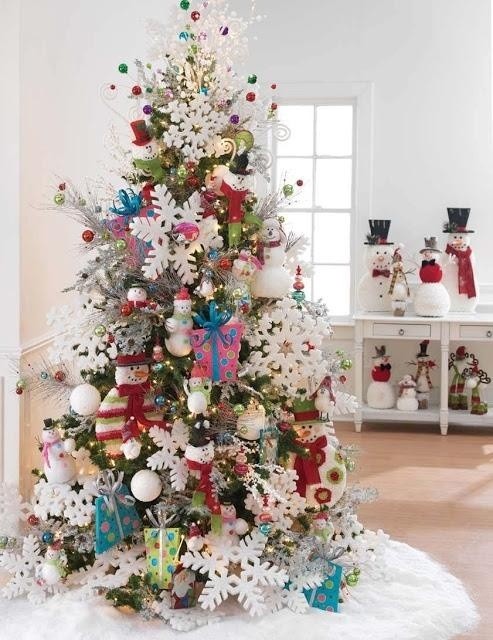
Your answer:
[353,313,493,435]
